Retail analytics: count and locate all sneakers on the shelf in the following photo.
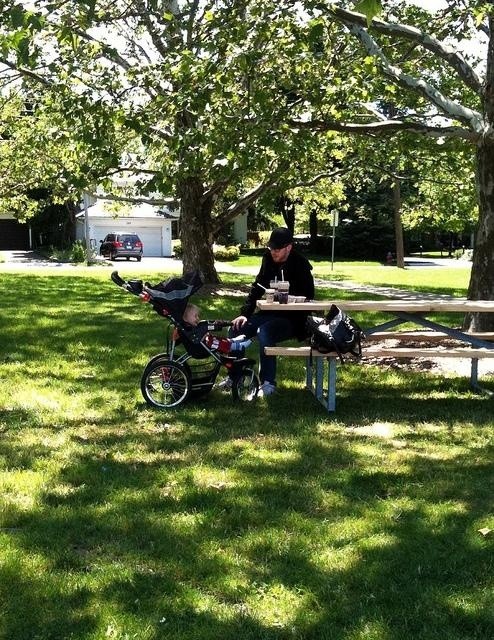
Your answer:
[246,380,277,401]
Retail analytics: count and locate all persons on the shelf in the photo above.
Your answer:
[210,227,314,402]
[181,302,254,359]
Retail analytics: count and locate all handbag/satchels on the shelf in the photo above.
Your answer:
[306,303,365,365]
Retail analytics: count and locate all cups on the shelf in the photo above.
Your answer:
[277,281,290,304]
[270,280,280,303]
[265,289,275,304]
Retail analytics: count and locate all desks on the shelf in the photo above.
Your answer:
[256,298,494,411]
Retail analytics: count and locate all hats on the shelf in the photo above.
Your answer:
[265,227,293,250]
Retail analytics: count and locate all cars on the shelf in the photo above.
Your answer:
[99,232,142,261]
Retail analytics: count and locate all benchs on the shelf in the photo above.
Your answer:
[263,332,494,411]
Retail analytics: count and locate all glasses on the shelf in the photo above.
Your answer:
[266,245,284,253]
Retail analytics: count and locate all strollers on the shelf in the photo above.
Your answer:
[111,271,259,408]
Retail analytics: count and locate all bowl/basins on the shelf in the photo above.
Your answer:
[294,296,307,303]
